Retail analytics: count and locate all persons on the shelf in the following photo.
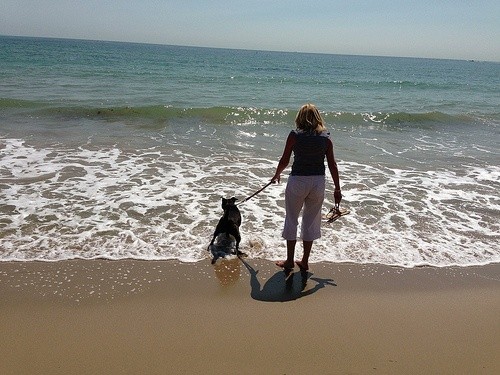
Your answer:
[270,104,342,270]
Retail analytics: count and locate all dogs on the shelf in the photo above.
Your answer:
[207,197,247,265]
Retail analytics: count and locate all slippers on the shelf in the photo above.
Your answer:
[326,209,350,223]
[320,207,347,220]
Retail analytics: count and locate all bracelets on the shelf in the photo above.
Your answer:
[334,189,340,192]
